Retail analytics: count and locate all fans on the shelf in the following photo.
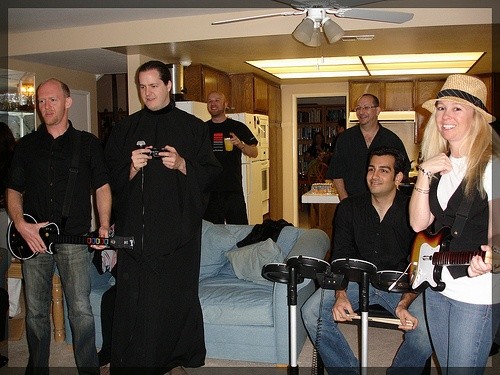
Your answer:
[211,0,414,29]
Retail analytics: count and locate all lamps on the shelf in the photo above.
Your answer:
[180,60,192,94]
[293,8,343,48]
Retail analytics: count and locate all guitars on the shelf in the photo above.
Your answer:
[6,214,135,260]
[408,226,500,295]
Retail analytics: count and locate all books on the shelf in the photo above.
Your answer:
[299,144,311,155]
[328,127,337,138]
[298,109,320,123]
[328,109,346,121]
[298,127,321,140]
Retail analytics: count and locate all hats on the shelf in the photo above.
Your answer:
[422,74,496,123]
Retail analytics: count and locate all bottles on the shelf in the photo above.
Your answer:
[311,183,336,195]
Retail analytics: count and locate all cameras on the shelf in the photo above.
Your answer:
[146,147,165,157]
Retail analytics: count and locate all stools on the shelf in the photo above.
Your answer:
[313,304,433,375]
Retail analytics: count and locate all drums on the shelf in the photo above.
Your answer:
[330,258,377,282]
[286,255,330,279]
[370,270,411,293]
[261,262,304,284]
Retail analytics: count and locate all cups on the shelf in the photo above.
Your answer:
[224,138,233,151]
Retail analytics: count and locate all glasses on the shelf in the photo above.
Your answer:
[354,105,378,111]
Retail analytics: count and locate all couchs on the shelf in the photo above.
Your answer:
[53,225,332,363]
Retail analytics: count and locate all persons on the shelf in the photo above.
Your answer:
[301,146,435,375]
[93,247,118,375]
[331,94,411,201]
[330,119,346,154]
[305,132,331,161]
[0,122,16,368]
[409,74,500,375]
[4,79,112,375]
[104,60,224,375]
[203,91,259,225]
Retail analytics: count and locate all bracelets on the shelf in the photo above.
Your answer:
[414,184,430,194]
[418,165,433,179]
[241,141,245,149]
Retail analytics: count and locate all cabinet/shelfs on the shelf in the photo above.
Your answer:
[297,104,347,183]
[184,63,284,223]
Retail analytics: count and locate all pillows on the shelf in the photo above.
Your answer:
[224,238,284,283]
[200,218,238,282]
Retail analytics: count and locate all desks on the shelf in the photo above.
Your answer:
[304,190,339,263]
